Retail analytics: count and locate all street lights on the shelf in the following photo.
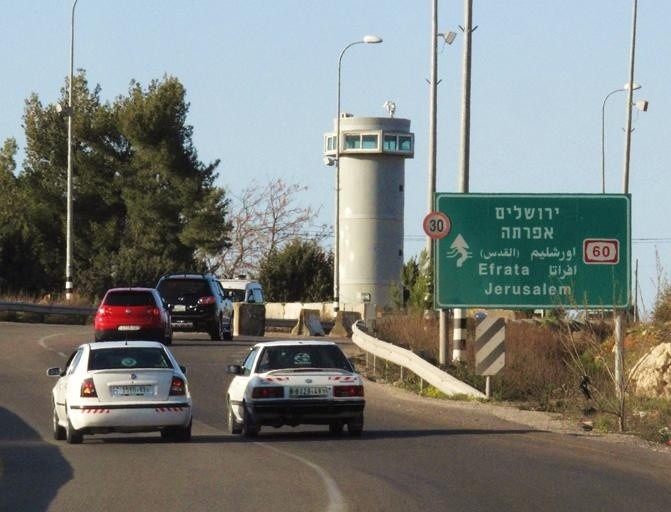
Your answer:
[332,35,382,316]
[600,83,641,194]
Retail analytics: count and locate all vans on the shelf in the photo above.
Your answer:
[156,272,263,340]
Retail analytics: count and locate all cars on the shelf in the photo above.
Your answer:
[45,339,193,445]
[94,287,173,344]
[225,339,365,437]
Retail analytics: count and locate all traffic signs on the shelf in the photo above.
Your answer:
[424,191,632,310]
